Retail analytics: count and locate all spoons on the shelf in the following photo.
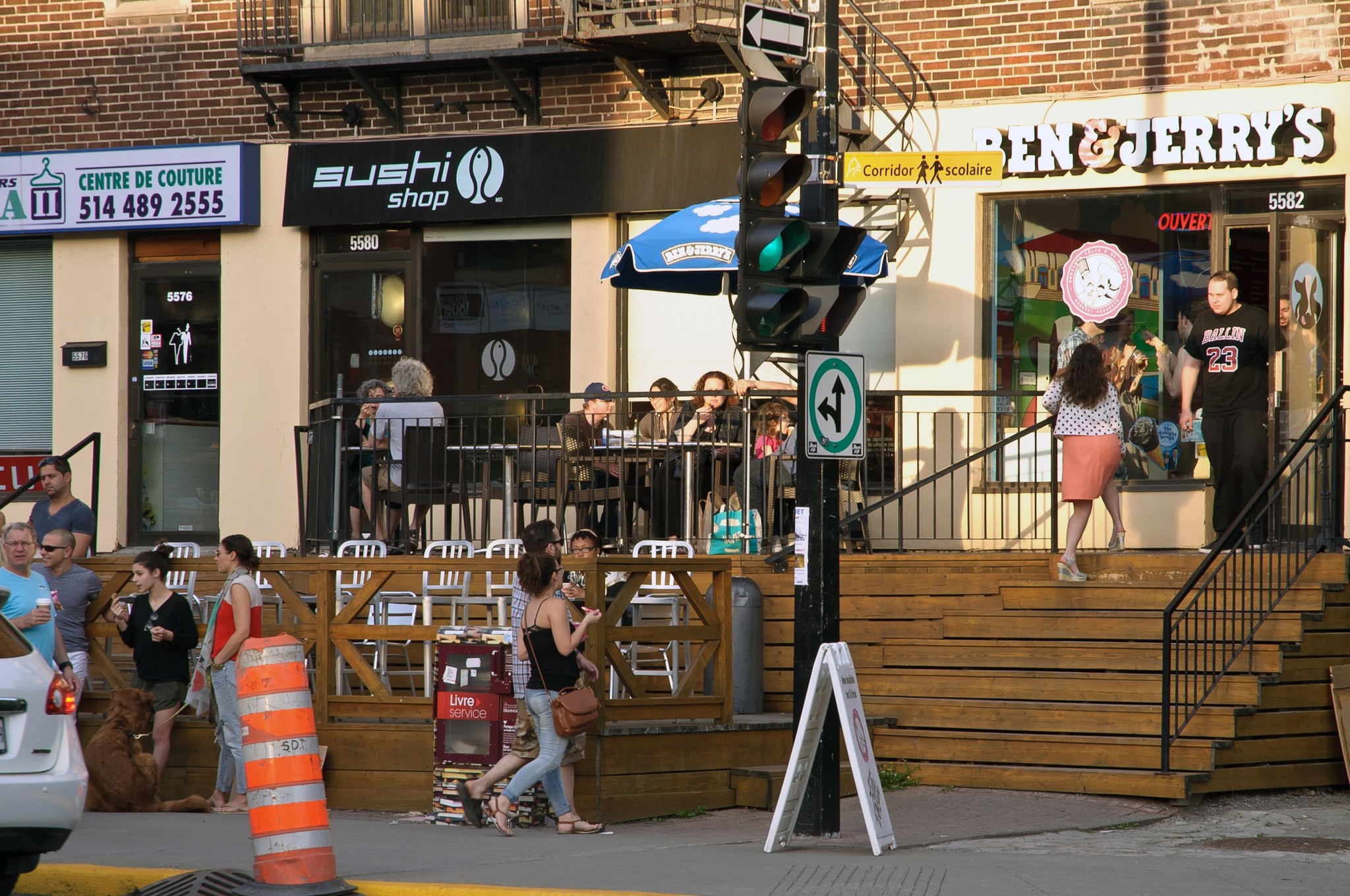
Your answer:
[582,607,595,611]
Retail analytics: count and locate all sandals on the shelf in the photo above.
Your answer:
[483,797,514,836]
[557,821,601,834]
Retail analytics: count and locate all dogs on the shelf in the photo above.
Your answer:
[83,685,216,813]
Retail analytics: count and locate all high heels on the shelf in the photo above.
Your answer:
[1108,525,1125,551]
[1057,554,1087,582]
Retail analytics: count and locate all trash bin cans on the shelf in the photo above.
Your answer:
[431,625,549,828]
[700,574,764,716]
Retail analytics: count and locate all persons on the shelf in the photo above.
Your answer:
[1041,269,1322,582]
[0,456,262,814]
[341,359,891,836]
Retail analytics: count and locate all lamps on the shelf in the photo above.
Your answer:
[618,79,723,102]
[263,104,363,126]
[376,272,404,329]
[433,93,531,115]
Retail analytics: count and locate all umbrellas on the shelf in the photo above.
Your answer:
[598,199,892,552]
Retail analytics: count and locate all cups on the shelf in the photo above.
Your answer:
[36,598,52,610]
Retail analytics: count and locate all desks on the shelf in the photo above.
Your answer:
[447,444,562,557]
[589,446,666,555]
[628,439,754,554]
[341,446,388,537]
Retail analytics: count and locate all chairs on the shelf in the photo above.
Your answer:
[485,423,875,555]
[125,541,697,698]
[370,424,473,555]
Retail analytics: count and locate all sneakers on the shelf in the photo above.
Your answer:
[1199,537,1244,553]
[1247,530,1269,550]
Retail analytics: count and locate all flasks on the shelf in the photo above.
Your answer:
[563,583,573,596]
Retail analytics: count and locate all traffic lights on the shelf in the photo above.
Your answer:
[784,214,868,352]
[732,79,814,352]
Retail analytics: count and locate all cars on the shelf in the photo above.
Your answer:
[1,613,90,896]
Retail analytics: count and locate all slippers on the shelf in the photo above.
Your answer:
[210,805,250,814]
[458,785,483,830]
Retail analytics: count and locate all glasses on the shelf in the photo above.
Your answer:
[544,538,565,549]
[38,457,68,472]
[216,549,232,558]
[571,544,598,556]
[648,395,662,401]
[5,541,33,548]
[550,565,564,579]
[593,398,615,405]
[144,612,159,632]
[40,544,70,553]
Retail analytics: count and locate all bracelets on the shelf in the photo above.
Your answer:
[210,659,225,670]
[58,661,73,671]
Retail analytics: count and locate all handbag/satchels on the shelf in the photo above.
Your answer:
[697,494,763,555]
[550,686,602,738]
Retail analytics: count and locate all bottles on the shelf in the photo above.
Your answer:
[631,419,642,442]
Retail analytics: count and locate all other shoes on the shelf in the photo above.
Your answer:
[408,529,419,551]
[350,537,366,552]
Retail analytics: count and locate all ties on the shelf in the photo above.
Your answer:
[1173,459,1176,469]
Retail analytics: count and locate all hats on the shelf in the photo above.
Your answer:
[584,383,614,402]
